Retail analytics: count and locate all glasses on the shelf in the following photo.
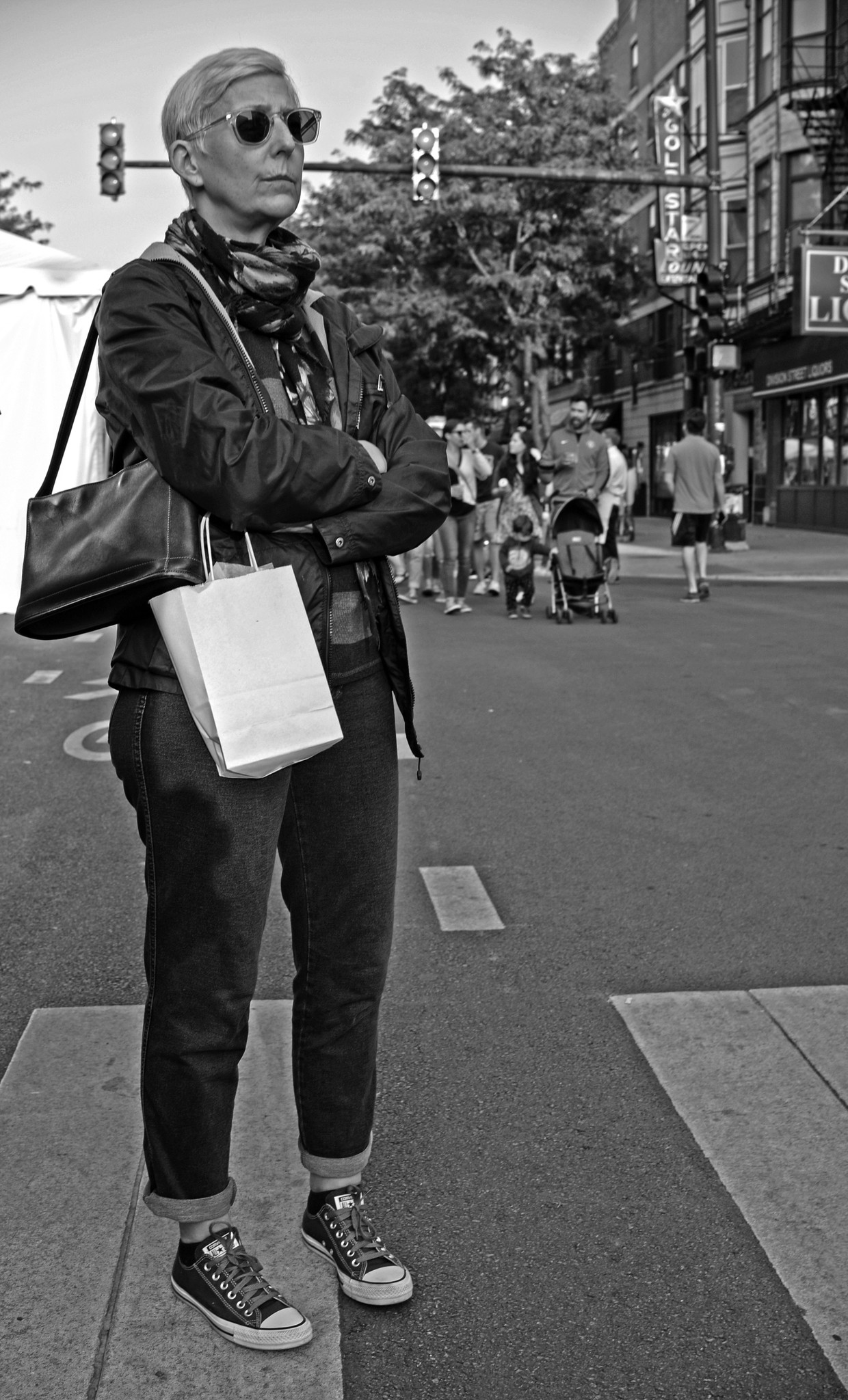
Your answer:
[449,432,462,435]
[183,108,322,147]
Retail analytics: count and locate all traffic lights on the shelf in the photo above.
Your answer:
[707,342,743,374]
[682,345,705,376]
[99,121,126,196]
[411,126,441,206]
[696,268,727,338]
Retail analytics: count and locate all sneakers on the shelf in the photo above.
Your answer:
[170,1221,313,1351]
[301,1180,413,1305]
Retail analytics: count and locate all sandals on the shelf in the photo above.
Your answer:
[393,571,409,584]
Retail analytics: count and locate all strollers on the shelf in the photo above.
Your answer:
[545,492,618,626]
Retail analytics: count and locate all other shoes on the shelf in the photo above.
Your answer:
[679,592,701,604]
[423,588,433,596]
[434,590,446,603]
[474,582,488,596]
[488,580,500,595]
[468,570,479,580]
[606,558,620,584]
[520,607,532,618]
[398,592,418,603]
[433,583,440,594]
[443,601,459,615]
[458,600,473,613]
[508,609,517,618]
[699,579,710,600]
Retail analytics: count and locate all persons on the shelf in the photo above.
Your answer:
[382,393,727,619]
[94,48,414,1350]
[783,457,831,486]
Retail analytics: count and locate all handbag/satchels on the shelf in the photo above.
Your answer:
[14,255,207,639]
[149,503,343,779]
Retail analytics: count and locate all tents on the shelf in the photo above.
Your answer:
[761,435,837,473]
[0,228,117,620]
